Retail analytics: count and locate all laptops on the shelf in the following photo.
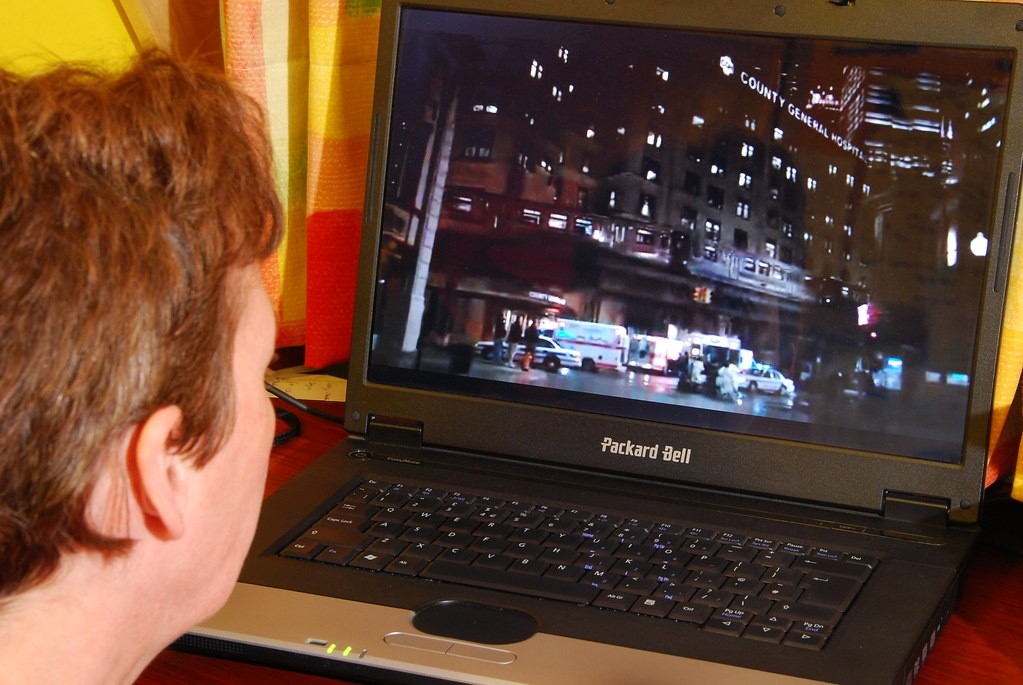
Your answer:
[171,0,1023,685]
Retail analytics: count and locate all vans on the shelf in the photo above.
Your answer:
[537,316,742,395]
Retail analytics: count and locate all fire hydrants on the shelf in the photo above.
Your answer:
[520,352,533,372]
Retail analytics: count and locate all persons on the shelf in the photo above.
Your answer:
[676,352,743,401]
[494,312,539,368]
[0,44,278,685]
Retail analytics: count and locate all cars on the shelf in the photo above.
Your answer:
[736,367,795,398]
[474,330,583,373]
